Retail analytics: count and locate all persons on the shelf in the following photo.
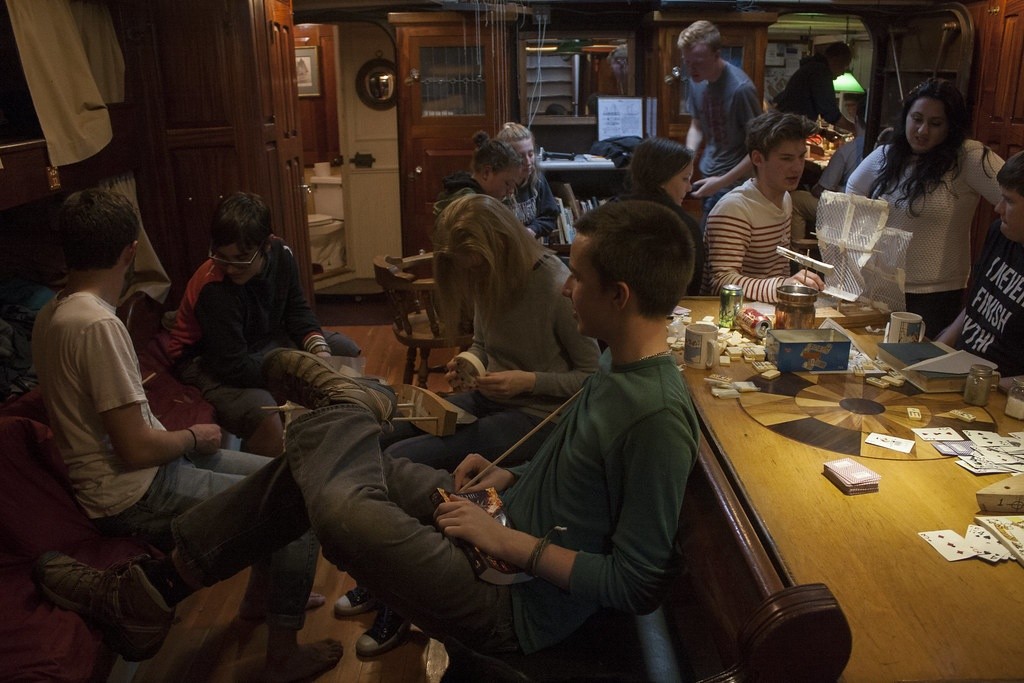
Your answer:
[845,77,1005,344]
[609,137,703,296]
[498,121,562,240]
[938,151,1024,378]
[675,20,761,236]
[771,41,856,142]
[30,188,345,683]
[789,90,865,254]
[432,129,520,322]
[701,113,825,302]
[607,43,629,82]
[168,192,331,459]
[34,200,699,683]
[333,195,601,657]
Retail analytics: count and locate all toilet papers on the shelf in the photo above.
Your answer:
[315,161,331,177]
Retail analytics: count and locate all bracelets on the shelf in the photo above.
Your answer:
[185,428,197,449]
[525,526,567,579]
[303,334,332,354]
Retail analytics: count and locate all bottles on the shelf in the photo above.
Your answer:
[1005,376,1024,420]
[773,286,819,330]
[823,135,854,151]
[964,364,993,407]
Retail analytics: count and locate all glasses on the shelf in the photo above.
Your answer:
[208,235,268,269]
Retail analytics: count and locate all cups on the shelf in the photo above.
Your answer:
[888,312,926,344]
[683,324,720,370]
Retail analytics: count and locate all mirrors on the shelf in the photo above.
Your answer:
[356,50,398,111]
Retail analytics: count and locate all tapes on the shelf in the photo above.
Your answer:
[454,352,486,387]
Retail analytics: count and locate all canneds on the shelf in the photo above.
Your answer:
[719,284,743,327]
[733,307,772,338]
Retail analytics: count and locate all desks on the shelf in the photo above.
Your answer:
[639,294,1024,683]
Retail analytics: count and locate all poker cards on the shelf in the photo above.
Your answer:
[910,427,964,441]
[864,432,916,454]
[918,524,1016,563]
[823,457,882,496]
[930,430,1024,476]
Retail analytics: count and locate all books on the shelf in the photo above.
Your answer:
[875,341,1000,394]
[428,488,533,586]
[549,180,608,245]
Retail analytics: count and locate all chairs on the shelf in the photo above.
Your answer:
[378,246,475,391]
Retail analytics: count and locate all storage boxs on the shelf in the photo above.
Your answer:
[761,327,850,373]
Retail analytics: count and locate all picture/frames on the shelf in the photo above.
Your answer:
[294,45,322,97]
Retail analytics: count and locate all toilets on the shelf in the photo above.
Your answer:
[309,175,347,268]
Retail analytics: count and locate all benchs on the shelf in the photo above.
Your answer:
[0,286,220,683]
[445,331,852,682]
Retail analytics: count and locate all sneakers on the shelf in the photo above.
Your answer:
[31,549,176,661]
[334,582,384,615]
[260,347,398,433]
[355,604,410,656]
